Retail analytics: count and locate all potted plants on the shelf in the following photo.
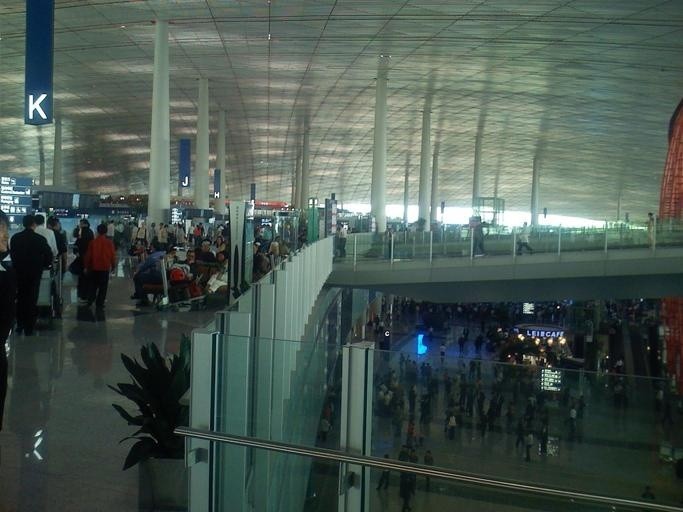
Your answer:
[107,331,192,512]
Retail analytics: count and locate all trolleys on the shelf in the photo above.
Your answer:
[1,254,64,319]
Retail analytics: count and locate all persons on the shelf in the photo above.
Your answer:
[517,222,532,255]
[337,224,352,257]
[321,294,664,511]
[2,211,13,426]
[645,213,654,248]
[8,210,291,336]
